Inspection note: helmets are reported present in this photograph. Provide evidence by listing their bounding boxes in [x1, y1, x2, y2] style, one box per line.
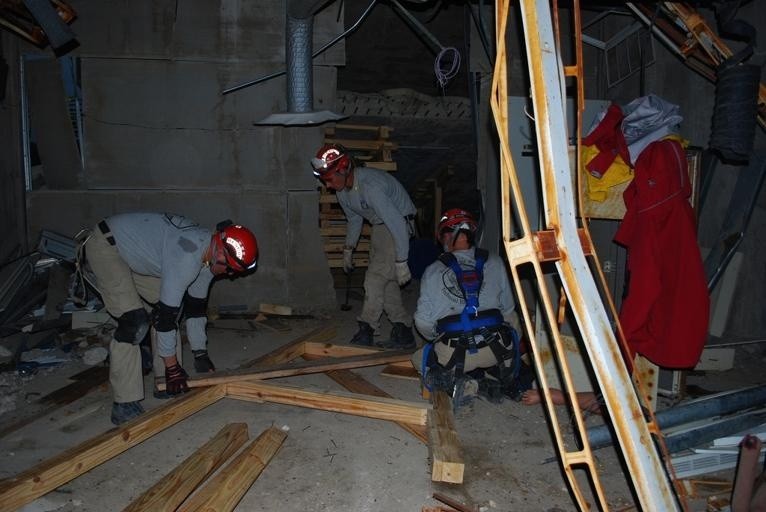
[311, 142, 351, 180]
[215, 224, 259, 276]
[435, 207, 479, 245]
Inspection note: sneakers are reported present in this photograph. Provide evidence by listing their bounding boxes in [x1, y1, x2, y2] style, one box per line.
[111, 401, 146, 425]
[451, 375, 481, 426]
[153, 383, 174, 399]
[477, 379, 505, 404]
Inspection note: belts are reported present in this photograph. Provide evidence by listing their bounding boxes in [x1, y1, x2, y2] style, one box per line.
[405, 213, 415, 222]
[97, 218, 116, 248]
[439, 334, 501, 350]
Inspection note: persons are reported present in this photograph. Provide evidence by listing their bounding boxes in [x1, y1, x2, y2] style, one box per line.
[522, 388, 605, 416]
[411, 207, 540, 406]
[75, 212, 259, 424]
[310, 142, 420, 353]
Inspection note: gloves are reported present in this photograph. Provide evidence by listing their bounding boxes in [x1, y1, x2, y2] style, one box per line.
[164, 362, 191, 396]
[342, 248, 355, 274]
[192, 354, 216, 374]
[394, 261, 412, 286]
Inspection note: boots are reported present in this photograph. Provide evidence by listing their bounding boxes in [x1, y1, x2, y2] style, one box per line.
[376, 321, 417, 351]
[349, 320, 376, 346]
